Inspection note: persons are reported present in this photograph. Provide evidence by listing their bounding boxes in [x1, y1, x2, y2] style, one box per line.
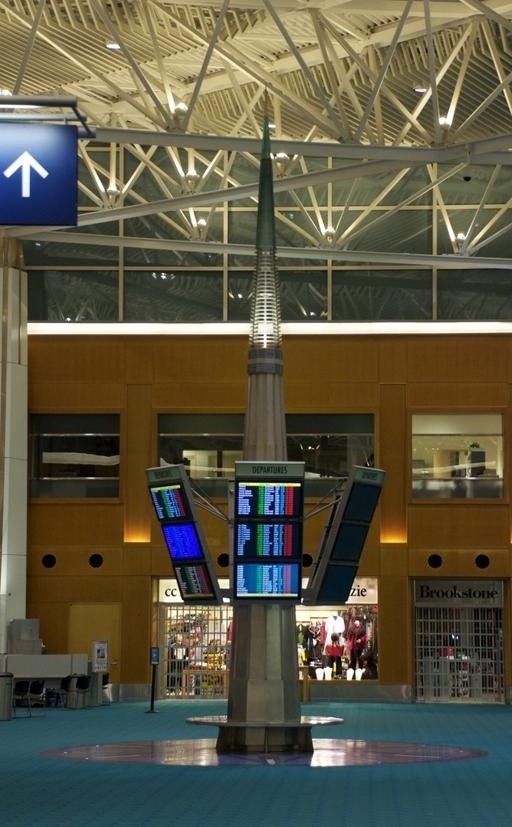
[326, 632, 345, 676]
[322, 613, 346, 668]
[348, 620, 367, 669]
[170, 630, 189, 692]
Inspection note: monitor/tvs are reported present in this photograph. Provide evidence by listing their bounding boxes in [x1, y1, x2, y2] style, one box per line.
[237, 480, 304, 521]
[235, 562, 300, 600]
[345, 483, 382, 523]
[331, 523, 369, 560]
[159, 521, 204, 563]
[169, 559, 215, 599]
[148, 483, 189, 521]
[318, 563, 358, 605]
[235, 523, 300, 559]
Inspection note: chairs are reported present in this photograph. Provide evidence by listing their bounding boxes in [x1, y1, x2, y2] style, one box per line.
[69, 677, 90, 709]
[11, 681, 32, 718]
[49, 677, 71, 708]
[28, 681, 44, 718]
[103, 673, 110, 705]
